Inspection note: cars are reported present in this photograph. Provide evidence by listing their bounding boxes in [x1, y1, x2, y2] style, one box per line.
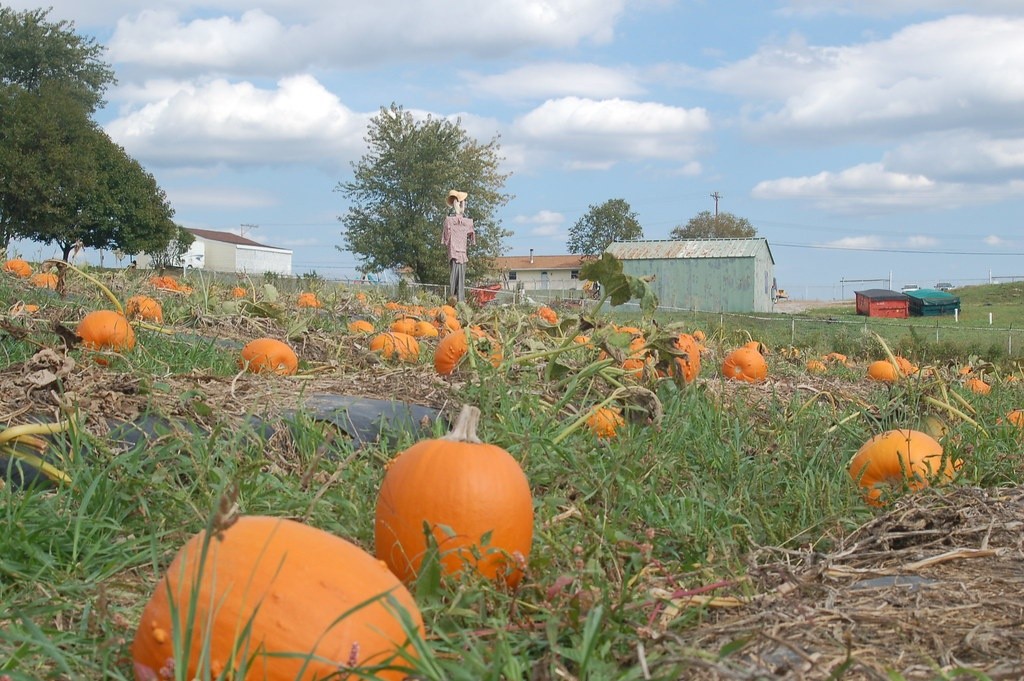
[935, 281, 956, 293]
[901, 284, 921, 295]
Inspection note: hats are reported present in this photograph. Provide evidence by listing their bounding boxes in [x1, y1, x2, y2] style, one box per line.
[445, 189, 468, 207]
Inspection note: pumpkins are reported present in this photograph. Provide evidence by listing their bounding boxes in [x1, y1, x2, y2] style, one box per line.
[132, 515, 424, 681]
[375, 404, 533, 597]
[1, 257, 1024, 513]
